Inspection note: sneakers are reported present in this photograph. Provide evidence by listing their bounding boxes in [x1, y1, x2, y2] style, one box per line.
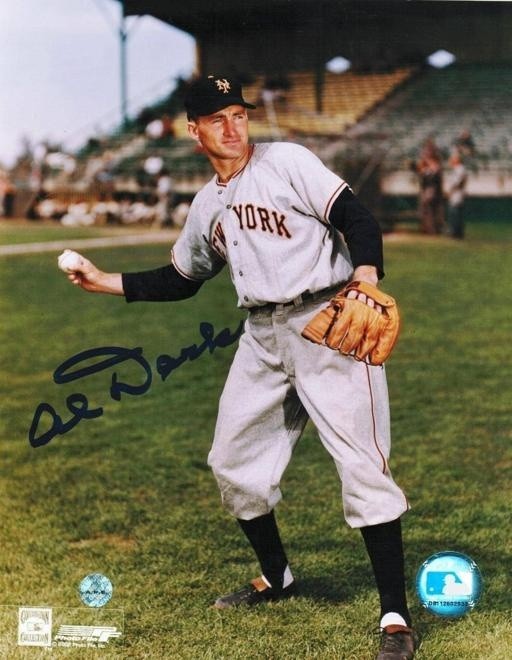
[375, 612, 420, 660]
[216, 575, 298, 612]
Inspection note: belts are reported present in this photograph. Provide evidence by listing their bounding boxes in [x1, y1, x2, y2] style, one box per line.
[247, 283, 348, 313]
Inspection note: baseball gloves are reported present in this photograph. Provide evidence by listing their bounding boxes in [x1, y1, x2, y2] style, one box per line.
[301, 280, 401, 366]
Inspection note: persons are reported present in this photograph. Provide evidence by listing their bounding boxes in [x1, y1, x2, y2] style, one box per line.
[0, 103, 467, 243]
[57, 73, 425, 660]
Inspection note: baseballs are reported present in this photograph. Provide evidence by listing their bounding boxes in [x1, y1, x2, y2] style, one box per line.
[57, 251, 81, 274]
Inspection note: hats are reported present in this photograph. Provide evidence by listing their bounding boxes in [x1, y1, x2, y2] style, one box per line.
[183, 72, 257, 122]
